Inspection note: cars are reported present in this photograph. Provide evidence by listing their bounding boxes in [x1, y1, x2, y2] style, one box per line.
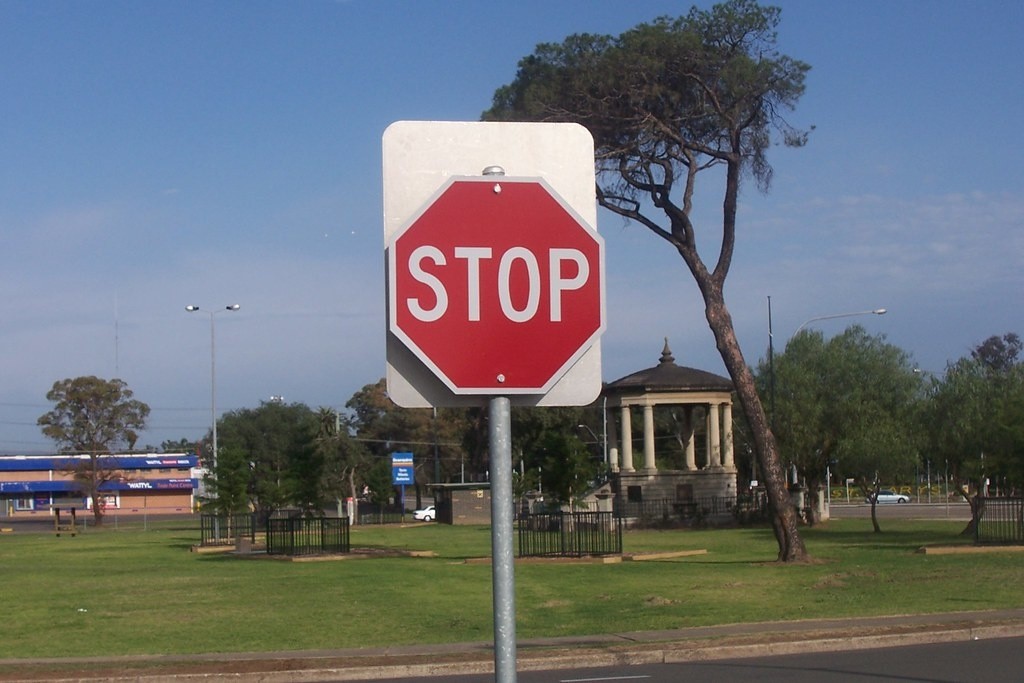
[412, 505, 436, 522]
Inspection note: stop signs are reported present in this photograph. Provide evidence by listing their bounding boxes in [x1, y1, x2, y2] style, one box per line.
[390, 172, 609, 397]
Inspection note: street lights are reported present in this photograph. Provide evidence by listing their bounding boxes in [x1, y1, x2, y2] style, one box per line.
[184, 303, 245, 547]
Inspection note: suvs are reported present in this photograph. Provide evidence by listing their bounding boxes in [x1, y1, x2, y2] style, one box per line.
[865, 490, 911, 503]
